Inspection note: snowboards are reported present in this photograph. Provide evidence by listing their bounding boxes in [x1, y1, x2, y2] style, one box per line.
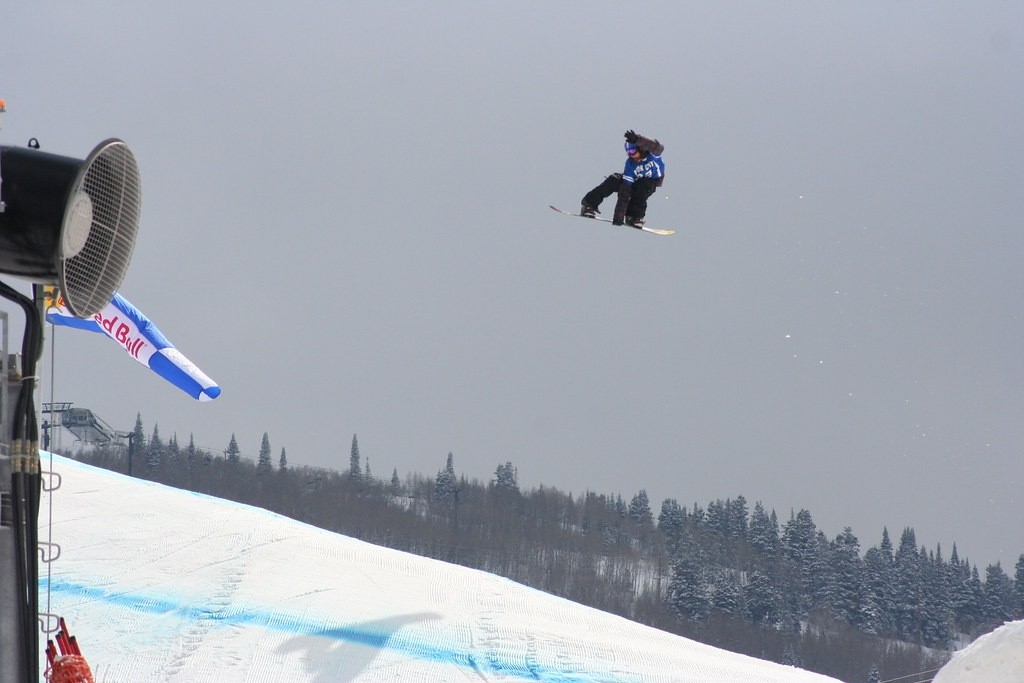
[549, 204, 676, 234]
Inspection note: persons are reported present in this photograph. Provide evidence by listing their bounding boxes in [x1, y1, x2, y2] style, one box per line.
[581, 130, 664, 229]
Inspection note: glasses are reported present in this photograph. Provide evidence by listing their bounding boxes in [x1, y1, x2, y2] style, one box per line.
[626, 149, 637, 155]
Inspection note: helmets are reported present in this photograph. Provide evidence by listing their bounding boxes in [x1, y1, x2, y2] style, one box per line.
[623, 138, 643, 151]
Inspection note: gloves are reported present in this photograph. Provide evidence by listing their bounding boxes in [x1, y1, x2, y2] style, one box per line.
[624, 130, 638, 143]
[613, 214, 624, 225]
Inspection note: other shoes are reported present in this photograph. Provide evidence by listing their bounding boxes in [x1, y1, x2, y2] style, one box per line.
[581, 206, 595, 218]
[626, 216, 644, 225]
